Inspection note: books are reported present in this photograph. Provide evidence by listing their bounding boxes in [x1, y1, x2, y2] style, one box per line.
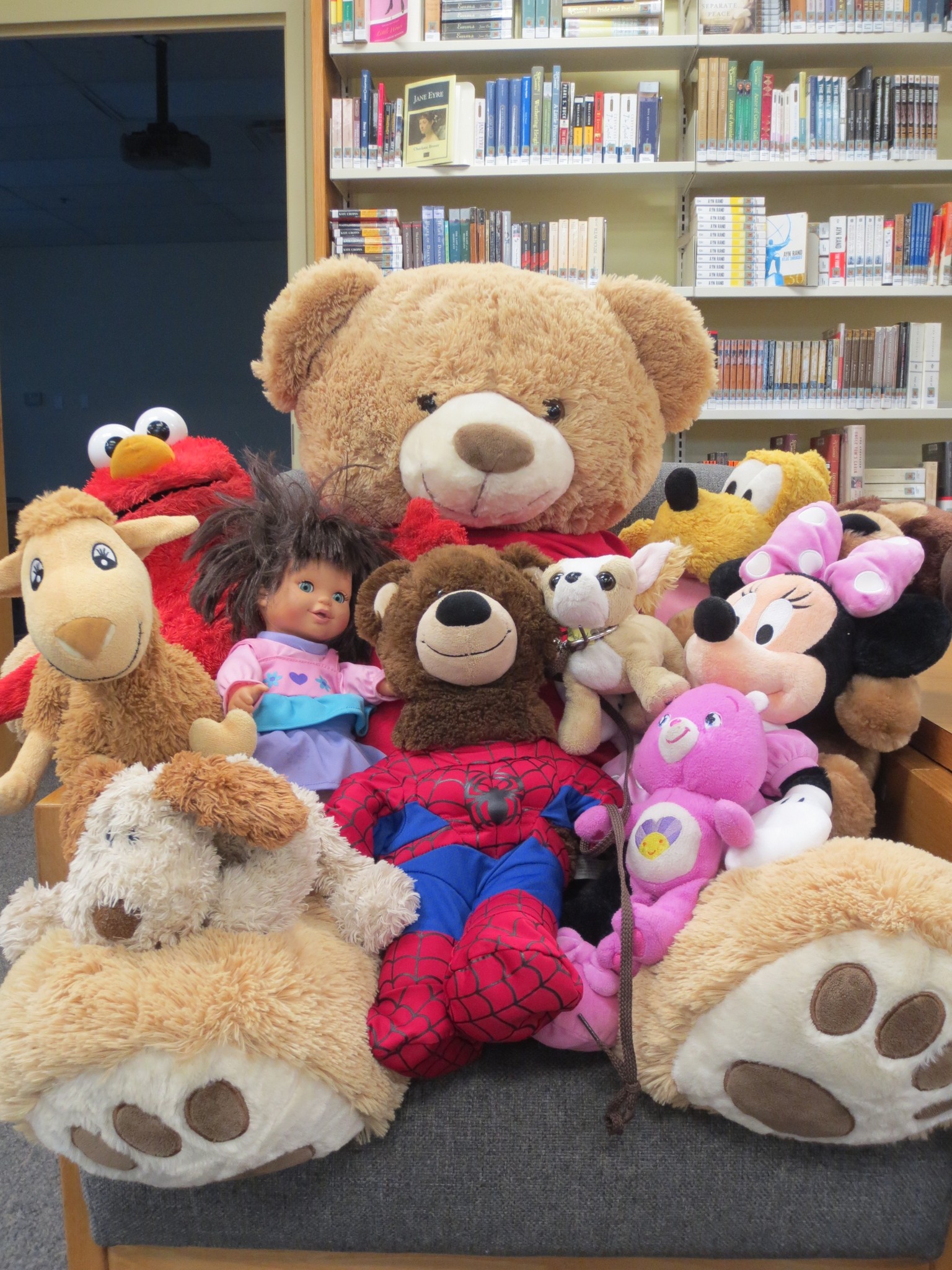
[682, 55, 941, 163]
[328, 0, 952, 43]
[325, 64, 663, 165]
[328, 205, 608, 284]
[687, 196, 952, 290]
[710, 321, 943, 408]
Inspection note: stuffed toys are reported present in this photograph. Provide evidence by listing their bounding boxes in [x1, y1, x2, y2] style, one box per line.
[0, 408, 952, 1192]
[250, 254, 713, 565]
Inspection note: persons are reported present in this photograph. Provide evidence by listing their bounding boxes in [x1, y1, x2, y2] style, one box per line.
[184, 452, 400, 801]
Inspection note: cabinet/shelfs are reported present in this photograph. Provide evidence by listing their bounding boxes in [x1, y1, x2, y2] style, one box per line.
[311, 0, 952, 505]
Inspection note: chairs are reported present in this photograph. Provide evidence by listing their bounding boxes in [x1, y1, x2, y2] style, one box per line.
[31, 723, 952, 1270]
